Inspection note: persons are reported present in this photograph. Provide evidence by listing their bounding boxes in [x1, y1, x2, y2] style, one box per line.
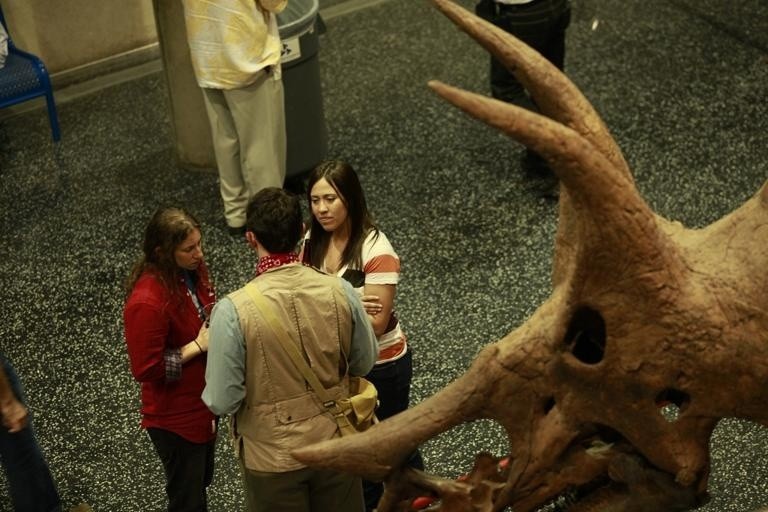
[0, 354, 64, 511]
[181, 0, 289, 237]
[299, 160, 426, 511]
[122, 205, 219, 512]
[202, 187, 380, 511]
[475, 1, 572, 190]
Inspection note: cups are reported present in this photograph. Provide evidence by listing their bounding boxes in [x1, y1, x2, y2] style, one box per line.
[201, 301, 217, 326]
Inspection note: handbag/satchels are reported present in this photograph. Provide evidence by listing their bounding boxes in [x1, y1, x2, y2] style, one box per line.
[330, 375, 381, 437]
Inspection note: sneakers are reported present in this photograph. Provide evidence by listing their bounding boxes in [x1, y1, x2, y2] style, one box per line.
[228, 225, 247, 236]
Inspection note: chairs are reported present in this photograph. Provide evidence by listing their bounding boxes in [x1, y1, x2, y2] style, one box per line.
[0, 2, 60, 143]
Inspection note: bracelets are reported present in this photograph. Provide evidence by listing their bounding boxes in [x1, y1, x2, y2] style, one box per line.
[194, 339, 203, 353]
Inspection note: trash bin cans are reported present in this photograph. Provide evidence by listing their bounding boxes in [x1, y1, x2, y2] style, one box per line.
[275, 0, 327, 178]
